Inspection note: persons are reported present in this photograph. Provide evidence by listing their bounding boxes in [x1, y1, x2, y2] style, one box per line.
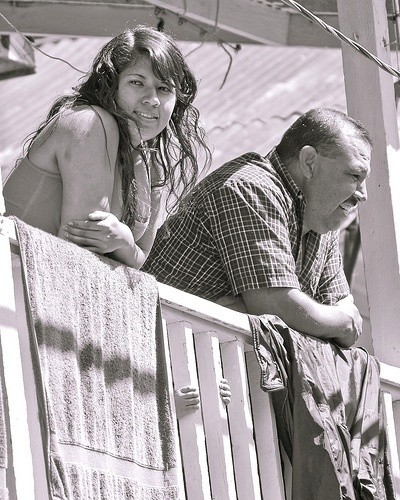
[140, 105, 373, 347]
[2, 28, 212, 270]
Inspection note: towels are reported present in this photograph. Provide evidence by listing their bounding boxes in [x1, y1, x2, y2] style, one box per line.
[8, 215, 181, 500]
